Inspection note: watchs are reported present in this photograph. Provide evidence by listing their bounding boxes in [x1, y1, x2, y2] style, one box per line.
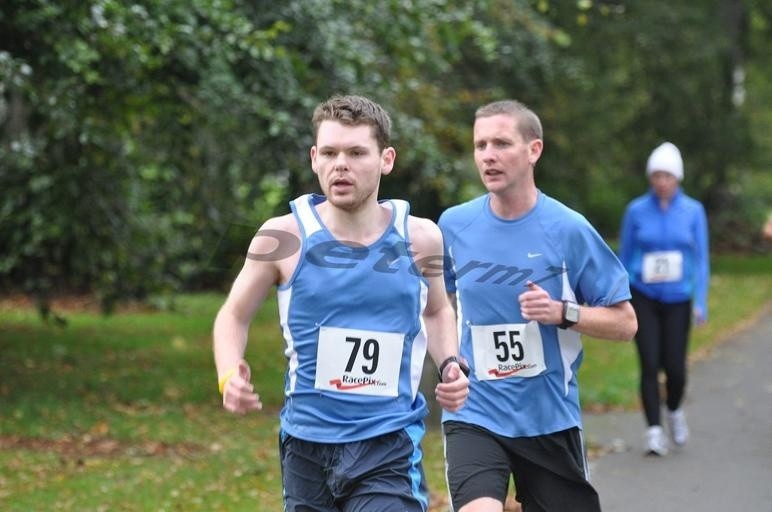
[559, 297, 580, 330]
[437, 357, 471, 383]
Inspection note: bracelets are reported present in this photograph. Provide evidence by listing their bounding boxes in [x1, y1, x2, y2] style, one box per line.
[218, 370, 238, 395]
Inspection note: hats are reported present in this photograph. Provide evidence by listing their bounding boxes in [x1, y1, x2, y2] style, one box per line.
[645, 141, 686, 184]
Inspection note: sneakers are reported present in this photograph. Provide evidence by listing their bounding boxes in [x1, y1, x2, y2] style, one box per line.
[641, 423, 670, 458]
[666, 405, 691, 445]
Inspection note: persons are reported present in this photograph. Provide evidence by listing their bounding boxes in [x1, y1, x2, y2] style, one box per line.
[619, 142, 710, 456]
[212, 94, 471, 512]
[432, 98, 639, 512]
